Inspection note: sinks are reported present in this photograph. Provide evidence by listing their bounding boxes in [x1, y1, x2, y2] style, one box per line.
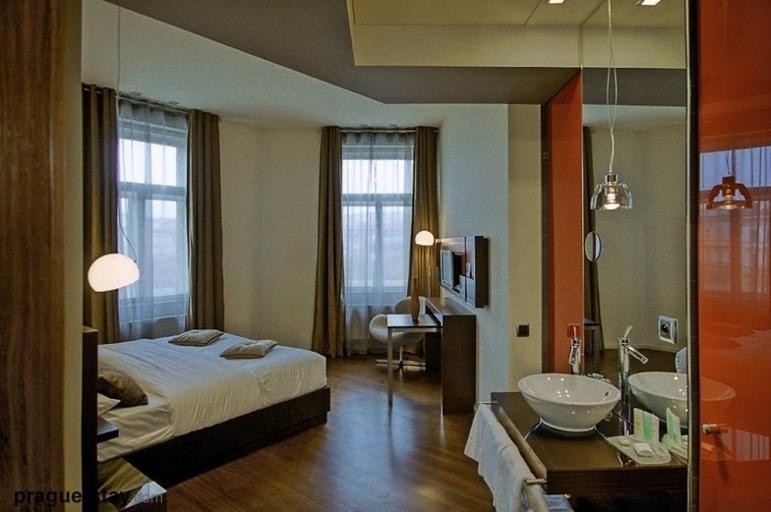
[626, 370, 737, 427]
[516, 372, 622, 431]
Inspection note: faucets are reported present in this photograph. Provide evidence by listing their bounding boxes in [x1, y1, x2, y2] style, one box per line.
[616, 324, 649, 406]
[567, 326, 582, 375]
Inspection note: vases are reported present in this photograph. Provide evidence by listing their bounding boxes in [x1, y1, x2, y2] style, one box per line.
[410, 278, 421, 322]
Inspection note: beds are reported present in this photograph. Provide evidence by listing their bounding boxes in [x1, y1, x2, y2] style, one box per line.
[82, 326, 331, 489]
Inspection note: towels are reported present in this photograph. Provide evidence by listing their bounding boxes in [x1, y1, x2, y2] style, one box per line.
[463, 404, 547, 512]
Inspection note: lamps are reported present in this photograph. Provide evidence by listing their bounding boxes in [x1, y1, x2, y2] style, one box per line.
[87, 7, 140, 293]
[415, 230, 465, 248]
[590, 0, 633, 211]
[705, 0, 754, 211]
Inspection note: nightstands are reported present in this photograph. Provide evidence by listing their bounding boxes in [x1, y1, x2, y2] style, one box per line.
[97, 457, 167, 512]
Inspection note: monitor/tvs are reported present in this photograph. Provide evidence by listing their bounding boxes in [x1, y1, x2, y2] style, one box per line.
[440, 250, 462, 290]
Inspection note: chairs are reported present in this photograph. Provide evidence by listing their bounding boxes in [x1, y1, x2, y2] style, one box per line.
[368, 296, 426, 377]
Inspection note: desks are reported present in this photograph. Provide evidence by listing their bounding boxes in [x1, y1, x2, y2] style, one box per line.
[424, 297, 477, 415]
[386, 314, 440, 407]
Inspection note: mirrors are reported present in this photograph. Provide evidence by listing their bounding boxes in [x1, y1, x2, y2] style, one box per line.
[582, 0, 690, 462]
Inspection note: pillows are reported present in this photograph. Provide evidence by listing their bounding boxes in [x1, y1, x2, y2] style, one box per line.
[219, 339, 280, 358]
[167, 328, 224, 345]
[97, 364, 148, 407]
[97, 392, 121, 417]
